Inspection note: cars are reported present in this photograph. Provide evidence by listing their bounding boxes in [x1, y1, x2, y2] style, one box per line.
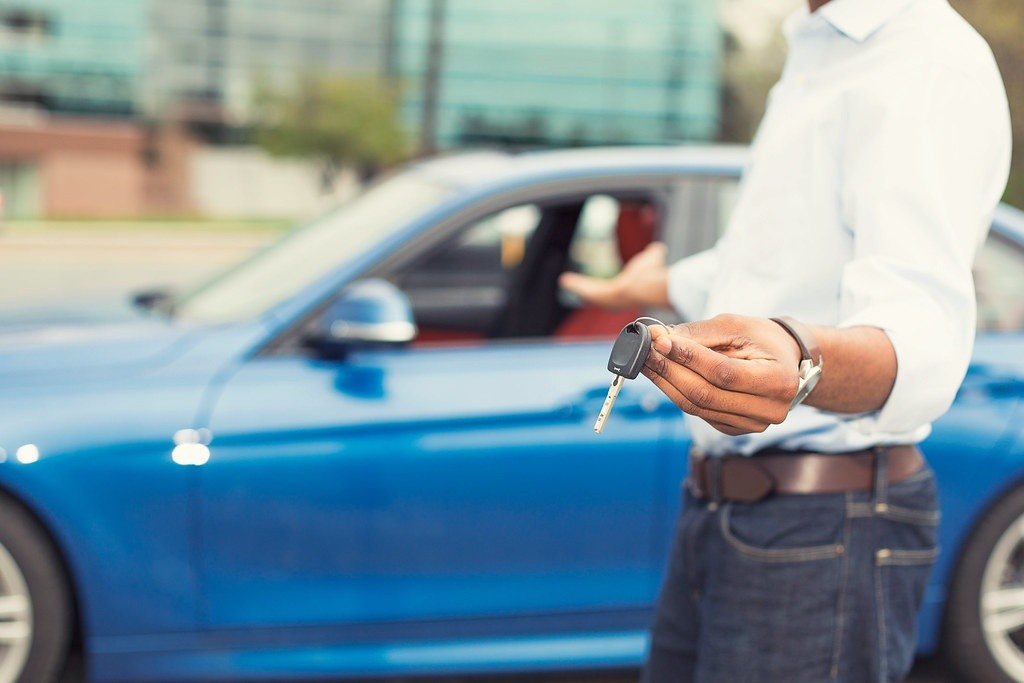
[1, 139, 1024, 683]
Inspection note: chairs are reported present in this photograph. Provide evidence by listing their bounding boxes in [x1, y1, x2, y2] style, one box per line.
[555, 202, 658, 336]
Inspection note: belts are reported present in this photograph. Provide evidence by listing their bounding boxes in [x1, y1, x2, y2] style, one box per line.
[690, 444, 924, 504]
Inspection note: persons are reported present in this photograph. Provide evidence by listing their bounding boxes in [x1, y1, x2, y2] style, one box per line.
[555, 1, 1013, 683]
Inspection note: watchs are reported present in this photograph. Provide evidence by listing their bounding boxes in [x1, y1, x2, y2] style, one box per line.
[766, 316, 825, 412]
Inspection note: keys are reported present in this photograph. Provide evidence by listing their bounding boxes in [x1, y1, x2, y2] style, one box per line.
[595, 320, 653, 434]
